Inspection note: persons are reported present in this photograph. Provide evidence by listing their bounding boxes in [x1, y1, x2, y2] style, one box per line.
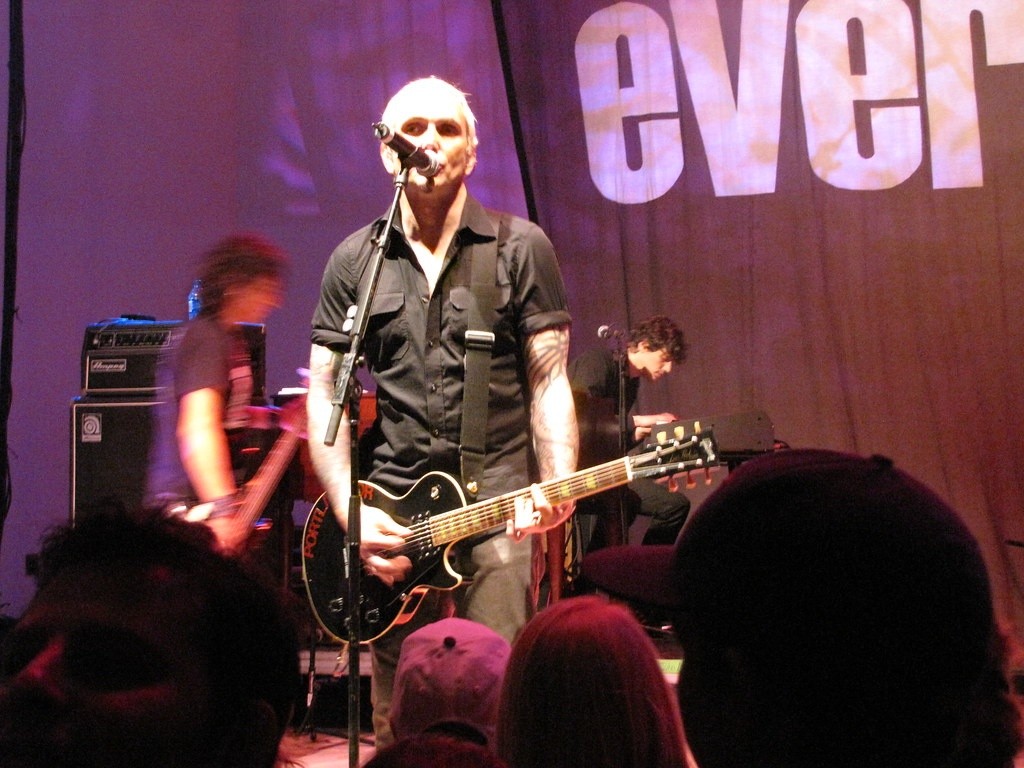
[566, 313, 694, 551]
[361, 595, 691, 767]
[583, 445, 1021, 767]
[0, 506, 301, 768]
[143, 232, 293, 554]
[305, 76, 580, 755]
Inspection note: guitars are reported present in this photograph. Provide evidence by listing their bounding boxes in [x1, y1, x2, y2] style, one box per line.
[218, 400, 306, 553]
[302, 419, 720, 647]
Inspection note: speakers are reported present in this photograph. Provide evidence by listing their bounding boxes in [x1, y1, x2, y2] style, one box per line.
[72, 396, 296, 594]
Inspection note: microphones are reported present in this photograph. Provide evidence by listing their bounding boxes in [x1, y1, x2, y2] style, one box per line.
[371, 122, 440, 177]
[597, 325, 613, 339]
